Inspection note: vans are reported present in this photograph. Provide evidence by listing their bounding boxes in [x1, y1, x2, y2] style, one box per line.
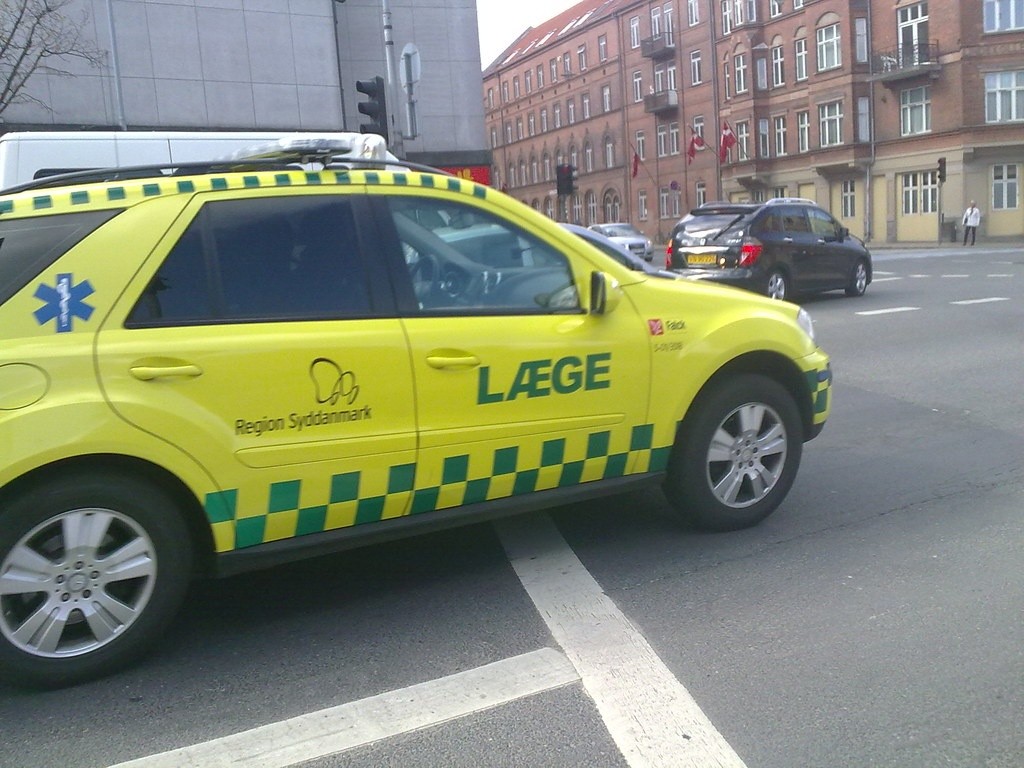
[1, 130, 451, 235]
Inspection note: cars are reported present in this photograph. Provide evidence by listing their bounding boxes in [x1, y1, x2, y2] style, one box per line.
[588, 221, 655, 262]
[414, 222, 678, 304]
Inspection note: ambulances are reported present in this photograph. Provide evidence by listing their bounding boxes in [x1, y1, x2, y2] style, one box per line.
[1, 148, 835, 692]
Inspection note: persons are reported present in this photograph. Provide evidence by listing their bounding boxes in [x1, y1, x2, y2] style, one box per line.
[962, 199, 981, 245]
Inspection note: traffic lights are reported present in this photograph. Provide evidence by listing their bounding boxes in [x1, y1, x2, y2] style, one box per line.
[935, 155, 947, 184]
[355, 73, 390, 146]
[557, 164, 575, 195]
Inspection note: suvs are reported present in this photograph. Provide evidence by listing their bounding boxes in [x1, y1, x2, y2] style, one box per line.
[664, 198, 874, 302]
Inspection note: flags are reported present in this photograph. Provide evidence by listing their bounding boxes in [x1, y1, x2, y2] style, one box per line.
[687, 128, 704, 165]
[633, 152, 640, 178]
[720, 121, 736, 162]
